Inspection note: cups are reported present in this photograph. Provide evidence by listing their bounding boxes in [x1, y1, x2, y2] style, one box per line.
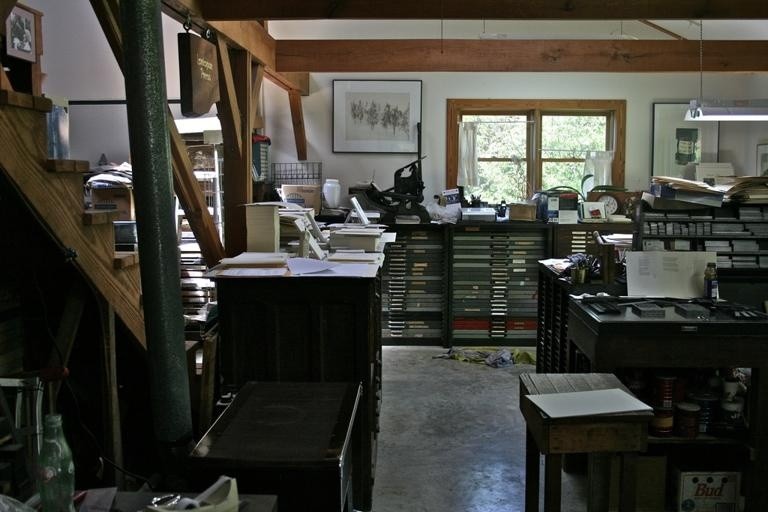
[572, 266, 591, 283]
[722, 378, 738, 400]
[592, 244, 615, 287]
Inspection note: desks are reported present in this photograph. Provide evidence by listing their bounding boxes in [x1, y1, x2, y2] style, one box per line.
[567, 288, 767, 511]
[20, 480, 284, 512]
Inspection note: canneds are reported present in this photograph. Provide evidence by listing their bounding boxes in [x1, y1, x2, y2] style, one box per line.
[626, 373, 718, 439]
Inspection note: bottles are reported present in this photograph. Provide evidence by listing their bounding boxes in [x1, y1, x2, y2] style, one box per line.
[703, 261, 718, 301]
[322, 178, 341, 209]
[36, 413, 77, 512]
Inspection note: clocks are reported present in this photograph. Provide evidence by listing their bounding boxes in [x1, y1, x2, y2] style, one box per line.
[598, 191, 621, 217]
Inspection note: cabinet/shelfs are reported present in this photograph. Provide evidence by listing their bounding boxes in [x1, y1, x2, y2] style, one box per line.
[380, 219, 448, 352]
[534, 250, 628, 379]
[551, 220, 639, 270]
[630, 195, 767, 282]
[448, 215, 550, 352]
[216, 247, 388, 511]
[168, 123, 228, 314]
[182, 375, 372, 509]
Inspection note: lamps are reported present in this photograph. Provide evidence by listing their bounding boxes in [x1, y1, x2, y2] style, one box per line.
[677, 16, 768, 123]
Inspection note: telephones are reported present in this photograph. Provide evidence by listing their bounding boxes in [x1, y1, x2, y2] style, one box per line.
[298, 229, 310, 259]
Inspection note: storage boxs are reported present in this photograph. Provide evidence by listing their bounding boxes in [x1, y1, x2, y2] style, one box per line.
[83, 180, 137, 222]
[507, 201, 540, 224]
[278, 181, 324, 216]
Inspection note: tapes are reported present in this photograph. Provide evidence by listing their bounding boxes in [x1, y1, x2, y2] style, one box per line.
[175, 494, 200, 510]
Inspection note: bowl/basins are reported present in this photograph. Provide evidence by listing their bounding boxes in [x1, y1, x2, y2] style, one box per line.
[509, 203, 538, 221]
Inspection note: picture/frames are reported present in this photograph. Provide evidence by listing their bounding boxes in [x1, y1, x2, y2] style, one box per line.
[648, 99, 722, 189]
[330, 76, 425, 156]
[756, 141, 767, 181]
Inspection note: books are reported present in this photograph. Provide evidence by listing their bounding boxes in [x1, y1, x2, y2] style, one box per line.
[523, 388, 653, 419]
[602, 175, 768, 269]
[177, 209, 383, 340]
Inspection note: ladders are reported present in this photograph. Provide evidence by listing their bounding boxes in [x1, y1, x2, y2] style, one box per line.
[173, 148, 225, 274]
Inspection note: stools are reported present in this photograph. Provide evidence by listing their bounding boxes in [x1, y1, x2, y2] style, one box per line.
[513, 373, 662, 510]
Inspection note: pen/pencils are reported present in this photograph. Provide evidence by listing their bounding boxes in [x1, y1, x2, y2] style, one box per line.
[574, 255, 599, 268]
[471, 194, 481, 201]
